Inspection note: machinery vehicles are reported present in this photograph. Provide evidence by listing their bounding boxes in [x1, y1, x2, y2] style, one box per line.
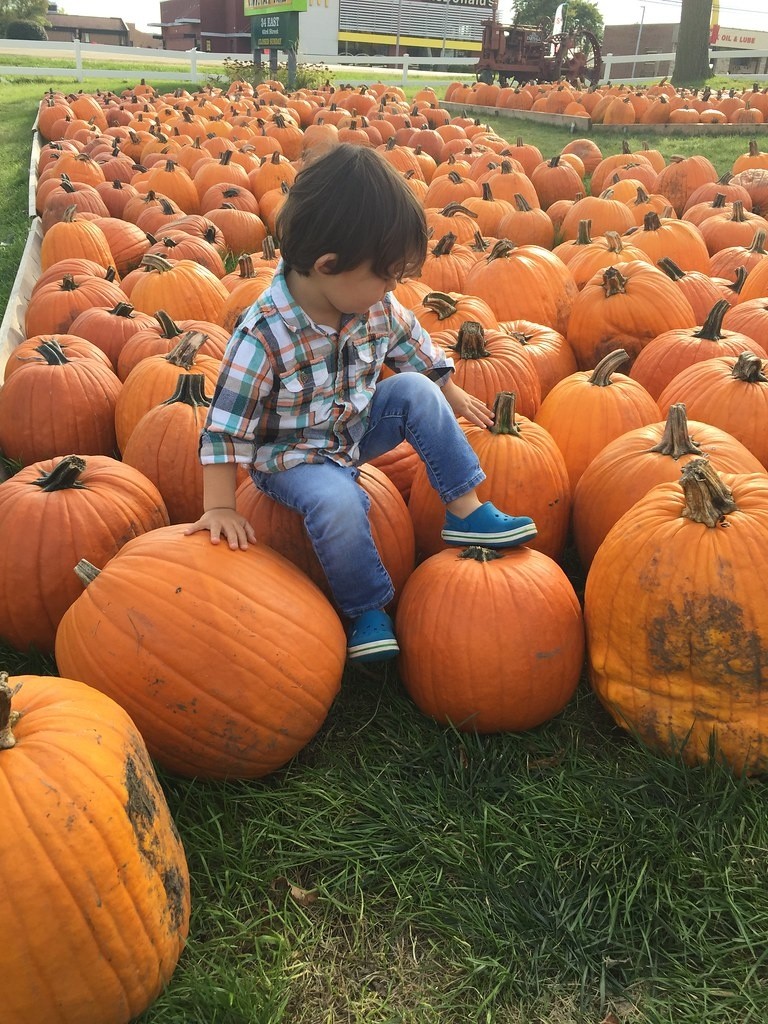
[474, 2, 603, 93]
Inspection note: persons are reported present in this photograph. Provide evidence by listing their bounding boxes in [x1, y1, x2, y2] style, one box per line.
[183, 140, 538, 664]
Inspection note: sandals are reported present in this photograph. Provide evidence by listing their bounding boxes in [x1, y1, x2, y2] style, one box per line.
[346, 609, 402, 664]
[441, 501, 541, 551]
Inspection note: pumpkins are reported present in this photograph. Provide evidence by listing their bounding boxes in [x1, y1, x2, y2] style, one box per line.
[0, 674, 192, 1024]
[444, 76, 768, 124]
[0, 139, 768, 781]
[26, 80, 544, 213]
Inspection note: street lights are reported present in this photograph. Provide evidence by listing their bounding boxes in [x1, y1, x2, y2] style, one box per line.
[628, 5, 647, 78]
